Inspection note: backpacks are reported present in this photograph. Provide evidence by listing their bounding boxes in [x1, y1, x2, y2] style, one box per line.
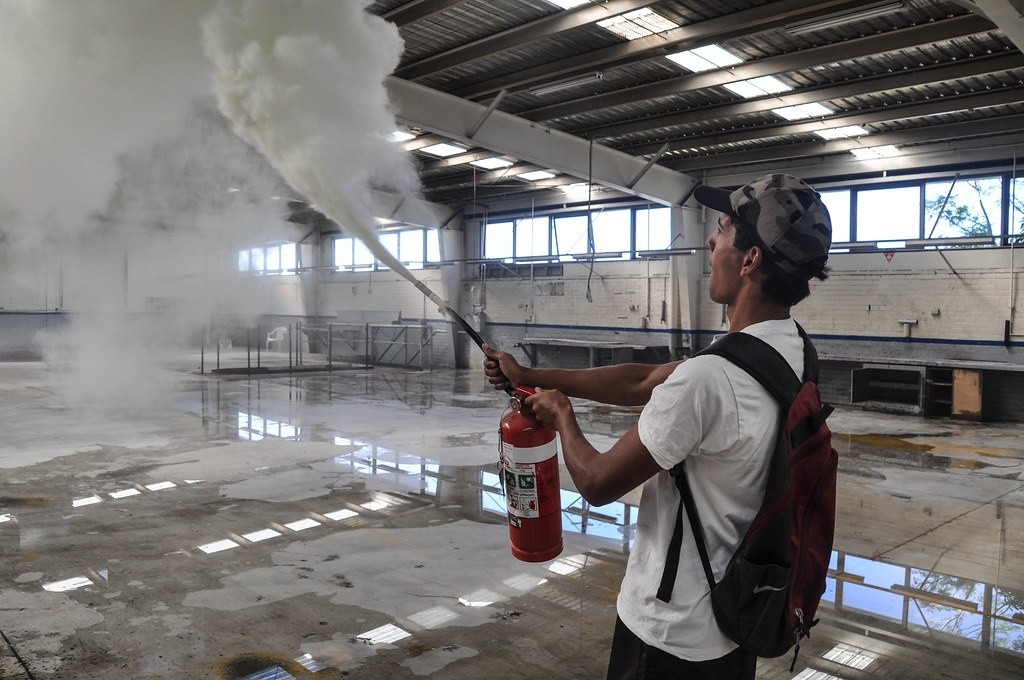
[655, 319, 839, 671]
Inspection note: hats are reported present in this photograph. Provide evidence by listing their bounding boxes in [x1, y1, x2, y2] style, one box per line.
[694, 173, 832, 280]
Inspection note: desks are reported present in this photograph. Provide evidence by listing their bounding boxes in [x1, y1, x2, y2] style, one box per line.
[818, 354, 1024, 423]
[501, 338, 646, 369]
[328, 323, 433, 371]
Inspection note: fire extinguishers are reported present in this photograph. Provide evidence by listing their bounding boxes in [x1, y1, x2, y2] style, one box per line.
[413, 279, 563, 562]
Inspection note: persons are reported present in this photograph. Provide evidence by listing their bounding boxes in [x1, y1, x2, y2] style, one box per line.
[481, 173, 833, 680]
